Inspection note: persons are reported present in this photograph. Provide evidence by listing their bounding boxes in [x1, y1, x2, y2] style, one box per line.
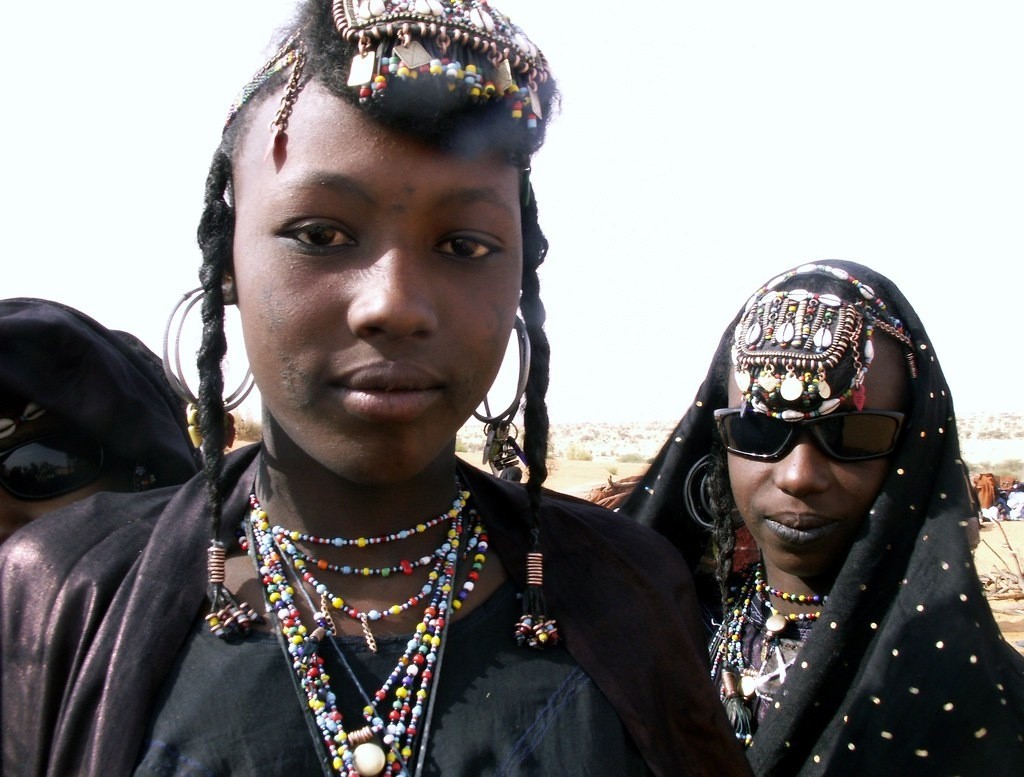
[619, 259, 1024, 776]
[0, 0, 750, 777]
[0, 301, 202, 543]
[980, 483, 1024, 522]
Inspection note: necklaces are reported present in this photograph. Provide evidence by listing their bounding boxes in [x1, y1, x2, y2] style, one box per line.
[240, 481, 488, 776]
[709, 563, 828, 743]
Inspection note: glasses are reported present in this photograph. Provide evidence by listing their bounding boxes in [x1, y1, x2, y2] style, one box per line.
[714, 408, 908, 464]
